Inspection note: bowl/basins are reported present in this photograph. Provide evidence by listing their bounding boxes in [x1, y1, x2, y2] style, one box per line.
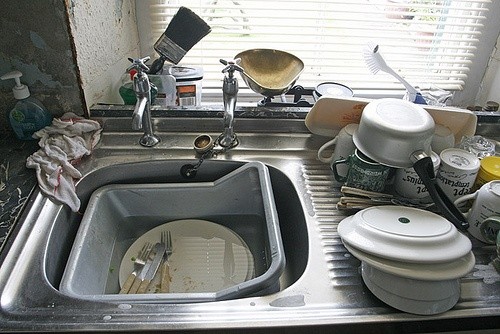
[361, 260, 460, 301]
[234, 49, 305, 96]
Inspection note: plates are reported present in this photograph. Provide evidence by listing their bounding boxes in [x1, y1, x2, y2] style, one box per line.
[337, 206, 473, 263]
[220, 225, 254, 288]
[359, 270, 460, 316]
[119, 219, 248, 294]
[340, 239, 475, 281]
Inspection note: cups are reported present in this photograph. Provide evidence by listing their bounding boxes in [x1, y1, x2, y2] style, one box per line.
[453, 179, 500, 244]
[480, 215, 500, 272]
[332, 147, 388, 197]
[317, 124, 358, 176]
[438, 146, 480, 209]
[431, 123, 456, 153]
[387, 150, 441, 199]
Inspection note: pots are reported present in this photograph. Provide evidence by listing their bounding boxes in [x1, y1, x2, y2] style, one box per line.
[352, 98, 469, 229]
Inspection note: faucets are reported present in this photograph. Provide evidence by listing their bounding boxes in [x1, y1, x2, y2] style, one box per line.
[125, 55, 160, 147]
[216, 56, 245, 149]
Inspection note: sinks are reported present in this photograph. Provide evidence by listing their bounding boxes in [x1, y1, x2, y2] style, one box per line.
[40, 157, 312, 313]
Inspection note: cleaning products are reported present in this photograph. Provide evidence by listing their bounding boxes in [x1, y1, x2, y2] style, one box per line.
[1, 70, 47, 139]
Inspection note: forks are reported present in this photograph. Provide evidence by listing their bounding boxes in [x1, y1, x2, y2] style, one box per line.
[160, 231, 172, 293]
[119, 242, 155, 294]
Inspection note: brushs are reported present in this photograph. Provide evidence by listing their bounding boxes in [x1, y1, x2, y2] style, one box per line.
[147, 5, 211, 74]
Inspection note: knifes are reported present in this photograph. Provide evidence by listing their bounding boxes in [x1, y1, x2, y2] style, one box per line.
[127, 243, 161, 294]
[137, 244, 165, 293]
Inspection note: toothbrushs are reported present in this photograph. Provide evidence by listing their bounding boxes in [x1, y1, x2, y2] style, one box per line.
[363, 42, 433, 104]
